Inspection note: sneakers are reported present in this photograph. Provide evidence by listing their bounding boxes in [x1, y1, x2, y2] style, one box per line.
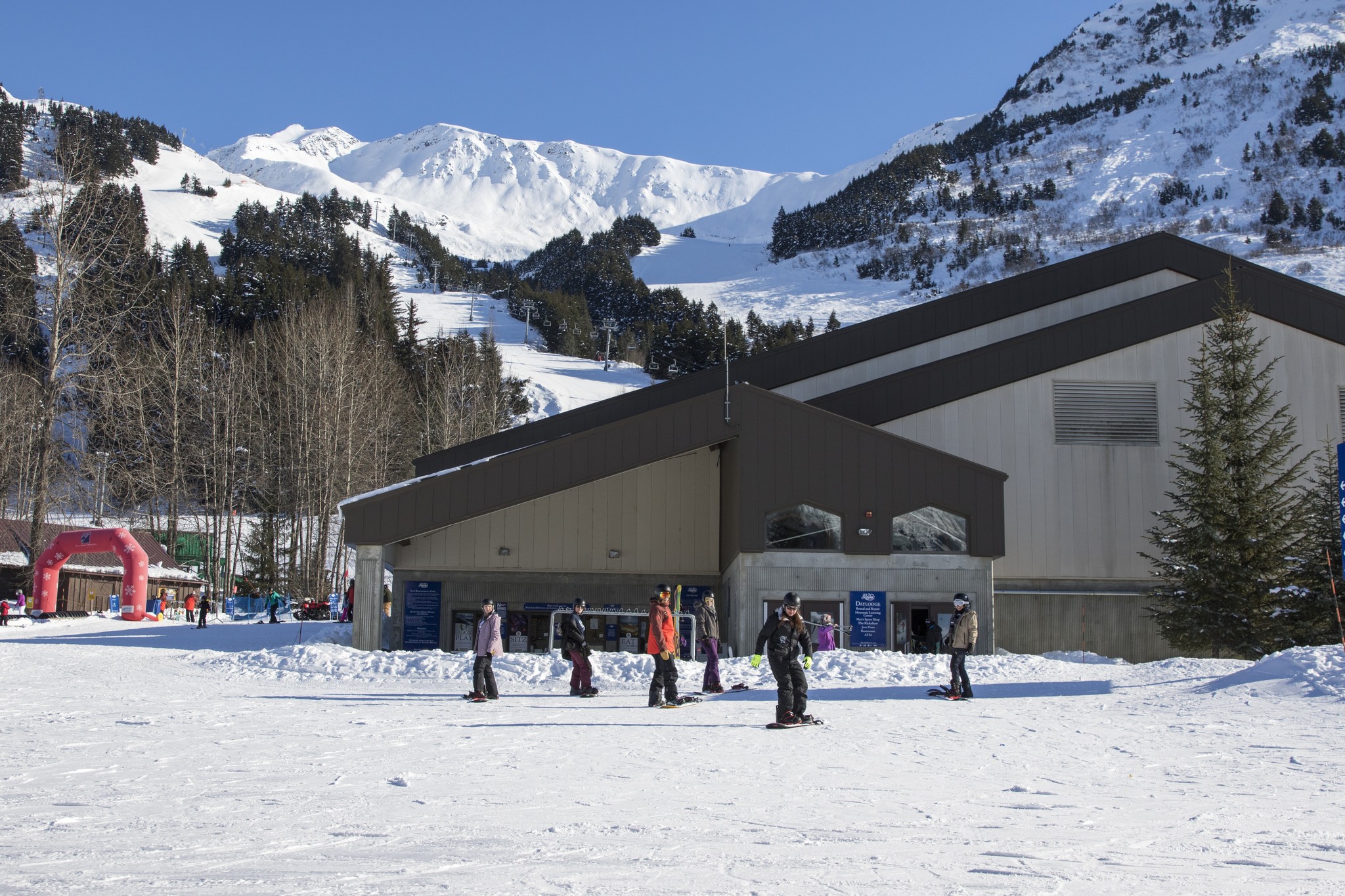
[570, 688, 581, 695]
[712, 682, 723, 692]
[779, 711, 802, 724]
[197, 625, 202, 629]
[666, 697, 685, 706]
[800, 714, 814, 723]
[202, 625, 206, 628]
[702, 686, 712, 692]
[649, 701, 666, 707]
[469, 691, 499, 699]
[581, 686, 599, 695]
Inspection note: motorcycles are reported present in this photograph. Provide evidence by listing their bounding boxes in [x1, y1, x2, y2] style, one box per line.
[293, 598, 332, 621]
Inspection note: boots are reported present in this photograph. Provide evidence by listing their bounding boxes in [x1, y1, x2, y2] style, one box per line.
[961, 680, 973, 698]
[949, 680, 961, 696]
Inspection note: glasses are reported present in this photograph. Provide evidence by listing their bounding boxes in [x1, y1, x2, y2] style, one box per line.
[704, 599, 713, 602]
[786, 605, 798, 610]
[953, 601, 969, 606]
[576, 605, 584, 609]
[659, 592, 670, 598]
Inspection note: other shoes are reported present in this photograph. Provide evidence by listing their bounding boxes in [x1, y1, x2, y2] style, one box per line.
[339, 620, 344, 622]
[269, 620, 277, 623]
[348, 621, 353, 622]
[0, 624, 4, 626]
[4, 624, 8, 626]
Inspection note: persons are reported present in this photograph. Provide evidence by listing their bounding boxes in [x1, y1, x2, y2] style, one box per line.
[197, 596, 211, 629]
[14, 589, 27, 615]
[472, 598, 503, 699]
[383, 584, 392, 617]
[157, 588, 168, 619]
[184, 590, 197, 623]
[817, 614, 836, 651]
[695, 591, 723, 693]
[923, 619, 944, 654]
[557, 597, 598, 696]
[345, 579, 355, 622]
[647, 584, 685, 706]
[945, 593, 978, 698]
[750, 592, 814, 725]
[340, 592, 349, 622]
[265, 587, 287, 623]
[0, 598, 10, 626]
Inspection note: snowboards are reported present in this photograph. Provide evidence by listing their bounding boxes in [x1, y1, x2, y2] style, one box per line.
[926, 689, 968, 701]
[765, 722, 815, 728]
[802, 619, 852, 637]
[463, 694, 487, 702]
[693, 688, 757, 695]
[660, 697, 704, 708]
[579, 689, 602, 698]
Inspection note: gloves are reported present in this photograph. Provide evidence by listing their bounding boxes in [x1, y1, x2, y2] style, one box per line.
[966, 642, 974, 656]
[802, 656, 812, 670]
[582, 641, 586, 648]
[265, 604, 267, 609]
[13, 605, 16, 609]
[750, 654, 761, 668]
[660, 650, 671, 660]
[944, 636, 951, 648]
[717, 640, 721, 650]
[282, 600, 287, 606]
[702, 635, 710, 648]
[485, 652, 492, 659]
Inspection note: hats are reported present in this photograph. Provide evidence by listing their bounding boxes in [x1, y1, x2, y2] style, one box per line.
[821, 613, 831, 623]
[161, 589, 166, 592]
[921, 616, 936, 627]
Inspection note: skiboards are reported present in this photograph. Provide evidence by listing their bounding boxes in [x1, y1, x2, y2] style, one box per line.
[191, 624, 209, 629]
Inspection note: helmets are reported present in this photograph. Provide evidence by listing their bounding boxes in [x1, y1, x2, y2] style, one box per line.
[573, 597, 586, 610]
[384, 584, 389, 588]
[702, 590, 715, 603]
[481, 598, 494, 610]
[783, 592, 801, 610]
[268, 587, 273, 592]
[350, 580, 354, 584]
[953, 593, 968, 605]
[16, 589, 22, 594]
[2, 598, 8, 603]
[654, 584, 671, 600]
